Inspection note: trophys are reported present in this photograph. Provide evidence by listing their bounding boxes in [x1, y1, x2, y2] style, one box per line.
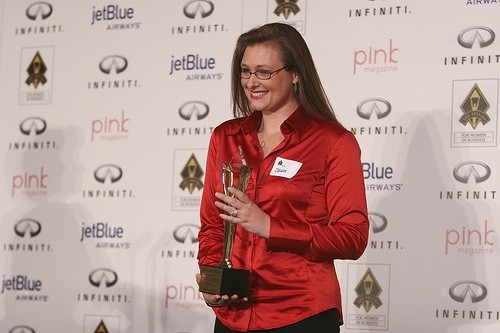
[198, 144, 251, 298]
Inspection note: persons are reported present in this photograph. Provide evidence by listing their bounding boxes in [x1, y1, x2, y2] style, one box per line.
[195, 23, 369, 333]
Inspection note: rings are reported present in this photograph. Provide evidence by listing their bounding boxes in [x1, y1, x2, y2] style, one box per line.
[233, 208, 238, 217]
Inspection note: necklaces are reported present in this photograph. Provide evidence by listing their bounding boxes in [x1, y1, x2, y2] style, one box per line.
[260, 117, 280, 148]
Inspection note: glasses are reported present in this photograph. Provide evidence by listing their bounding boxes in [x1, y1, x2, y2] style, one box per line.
[237, 66, 288, 80]
